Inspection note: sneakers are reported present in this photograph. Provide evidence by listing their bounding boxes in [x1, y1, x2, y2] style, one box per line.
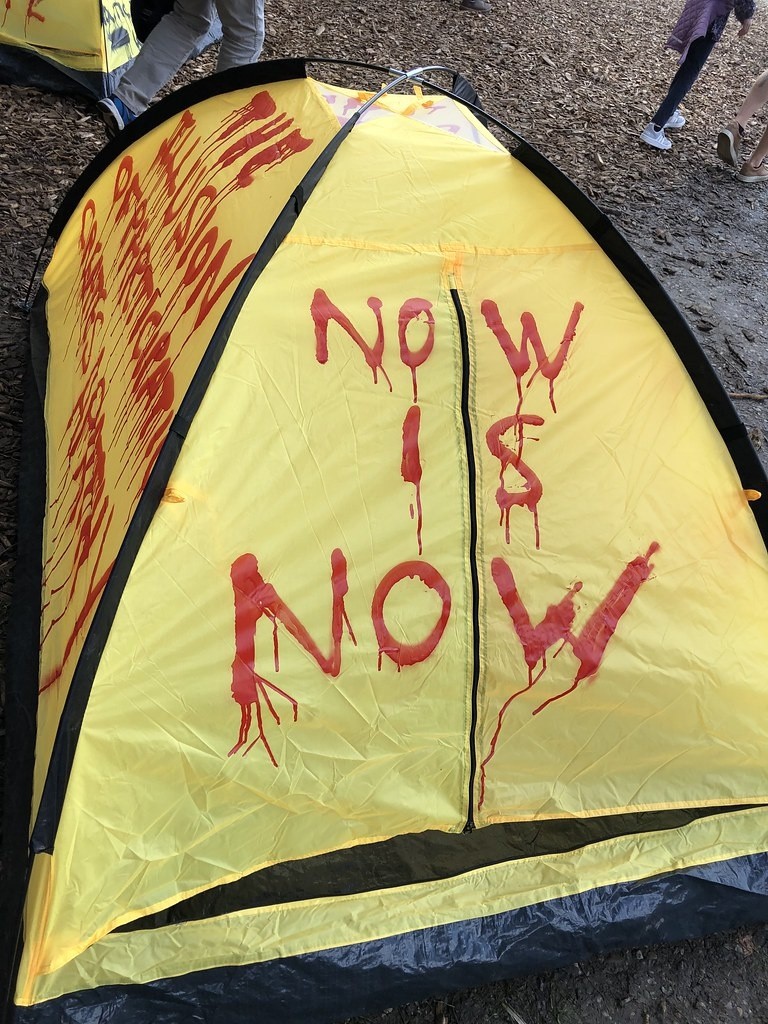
[661, 112, 687, 129]
[96, 95, 137, 141]
[639, 122, 674, 149]
[717, 120, 740, 167]
[736, 163, 768, 182]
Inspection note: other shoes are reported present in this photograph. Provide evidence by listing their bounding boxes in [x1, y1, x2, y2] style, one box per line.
[459, 0, 493, 12]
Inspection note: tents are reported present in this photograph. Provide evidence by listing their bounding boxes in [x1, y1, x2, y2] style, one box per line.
[0, 0, 223, 102]
[1, 57, 767, 1024]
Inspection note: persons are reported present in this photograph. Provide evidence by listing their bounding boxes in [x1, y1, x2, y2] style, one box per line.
[639, 0, 755, 150]
[95, 0, 267, 138]
[716, 68, 768, 183]
